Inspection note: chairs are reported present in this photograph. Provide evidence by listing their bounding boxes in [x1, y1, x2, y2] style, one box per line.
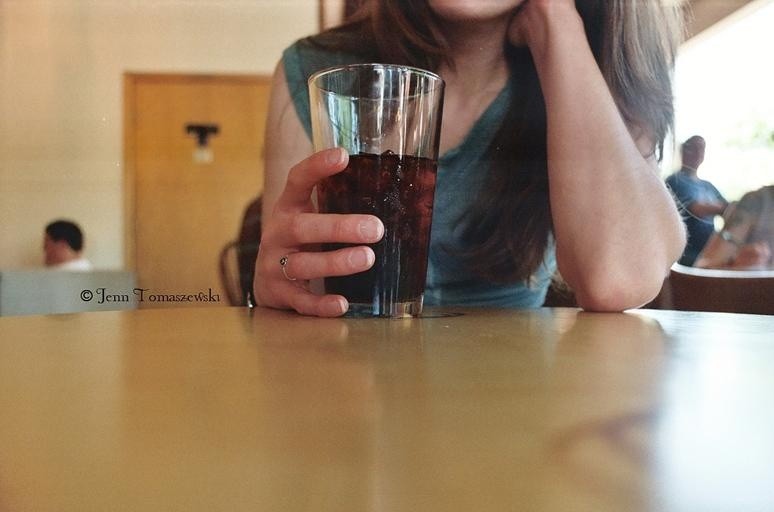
[669, 258, 773, 317]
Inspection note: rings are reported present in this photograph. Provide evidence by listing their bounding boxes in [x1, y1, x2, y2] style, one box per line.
[280, 251, 297, 282]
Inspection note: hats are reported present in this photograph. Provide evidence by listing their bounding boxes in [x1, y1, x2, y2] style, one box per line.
[682, 136, 705, 148]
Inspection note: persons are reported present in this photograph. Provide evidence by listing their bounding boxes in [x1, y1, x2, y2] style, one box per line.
[664, 136, 729, 266]
[42, 217, 92, 273]
[252, 0, 688, 317]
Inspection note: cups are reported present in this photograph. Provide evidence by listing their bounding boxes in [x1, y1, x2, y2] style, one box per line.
[304, 64, 445, 320]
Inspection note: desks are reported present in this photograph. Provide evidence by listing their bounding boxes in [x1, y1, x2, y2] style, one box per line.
[0, 307, 773, 512]
